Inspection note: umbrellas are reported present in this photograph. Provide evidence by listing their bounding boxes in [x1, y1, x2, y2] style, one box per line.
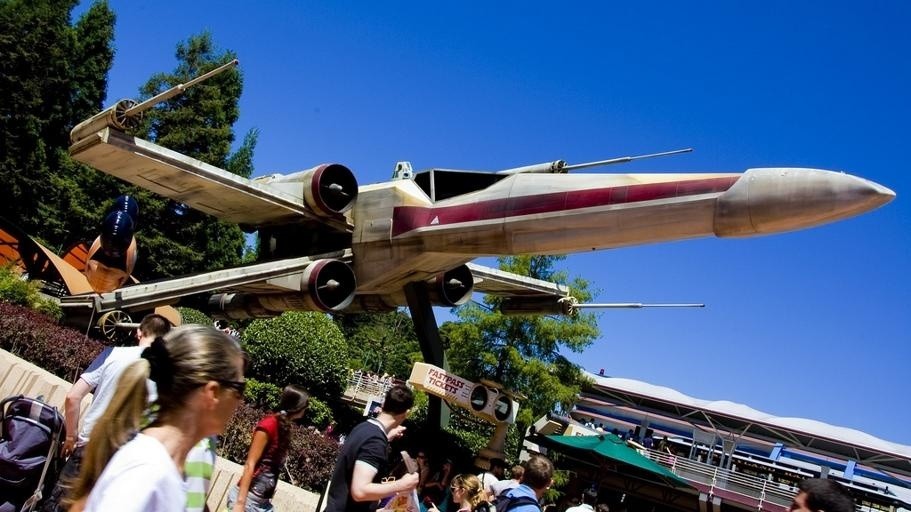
[542, 432, 700, 492]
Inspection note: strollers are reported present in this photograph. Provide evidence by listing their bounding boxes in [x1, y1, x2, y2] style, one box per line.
[0, 394, 69, 512]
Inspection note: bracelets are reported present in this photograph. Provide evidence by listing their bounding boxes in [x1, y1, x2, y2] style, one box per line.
[65, 434, 77, 441]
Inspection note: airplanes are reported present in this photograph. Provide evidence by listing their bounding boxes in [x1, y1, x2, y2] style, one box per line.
[26, 57, 899, 349]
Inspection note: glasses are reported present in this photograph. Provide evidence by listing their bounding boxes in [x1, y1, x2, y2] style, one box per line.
[219, 379, 247, 396]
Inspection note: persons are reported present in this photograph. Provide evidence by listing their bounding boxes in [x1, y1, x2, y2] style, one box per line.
[785, 477, 856, 512]
[346, 368, 397, 398]
[226, 384, 310, 512]
[322, 384, 420, 512]
[59, 322, 248, 512]
[697, 442, 807, 487]
[134, 404, 219, 512]
[577, 416, 672, 456]
[318, 448, 614, 512]
[44, 313, 172, 512]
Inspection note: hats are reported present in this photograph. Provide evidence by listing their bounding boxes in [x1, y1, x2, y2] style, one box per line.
[489, 457, 509, 469]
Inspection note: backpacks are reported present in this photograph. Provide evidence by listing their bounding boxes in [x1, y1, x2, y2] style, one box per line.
[471, 488, 539, 512]
